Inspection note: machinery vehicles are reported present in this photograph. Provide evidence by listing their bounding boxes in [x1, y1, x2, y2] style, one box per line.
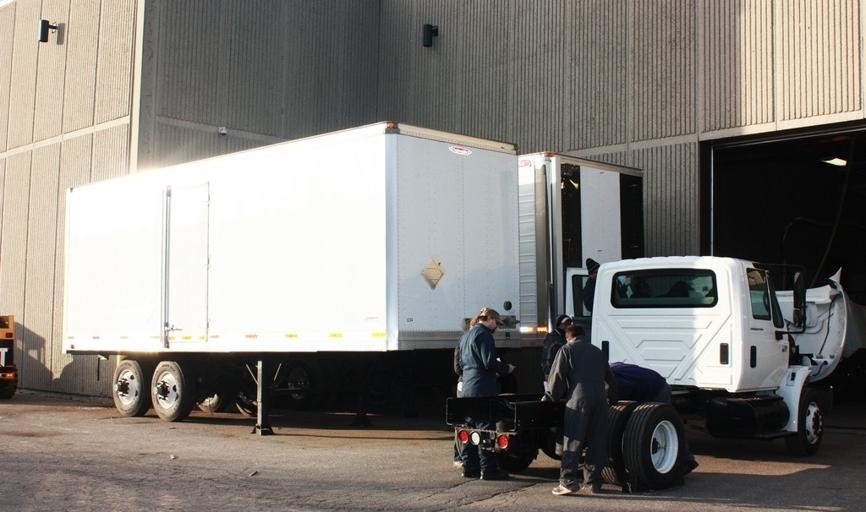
[0, 315, 19, 401]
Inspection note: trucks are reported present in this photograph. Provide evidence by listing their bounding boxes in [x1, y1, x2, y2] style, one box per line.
[443, 254, 830, 489]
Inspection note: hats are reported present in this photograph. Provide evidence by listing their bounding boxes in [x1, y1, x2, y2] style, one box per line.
[554, 313, 571, 329]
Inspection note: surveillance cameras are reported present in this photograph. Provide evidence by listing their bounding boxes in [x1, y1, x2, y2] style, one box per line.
[220, 127, 227, 136]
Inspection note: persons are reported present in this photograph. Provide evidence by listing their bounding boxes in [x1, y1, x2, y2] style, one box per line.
[541, 314, 573, 381]
[459, 308, 519, 481]
[451, 316, 472, 462]
[583, 257, 620, 315]
[541, 324, 619, 497]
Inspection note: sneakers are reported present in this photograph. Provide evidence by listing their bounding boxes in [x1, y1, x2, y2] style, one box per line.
[581, 483, 601, 494]
[552, 484, 571, 495]
[461, 470, 508, 480]
